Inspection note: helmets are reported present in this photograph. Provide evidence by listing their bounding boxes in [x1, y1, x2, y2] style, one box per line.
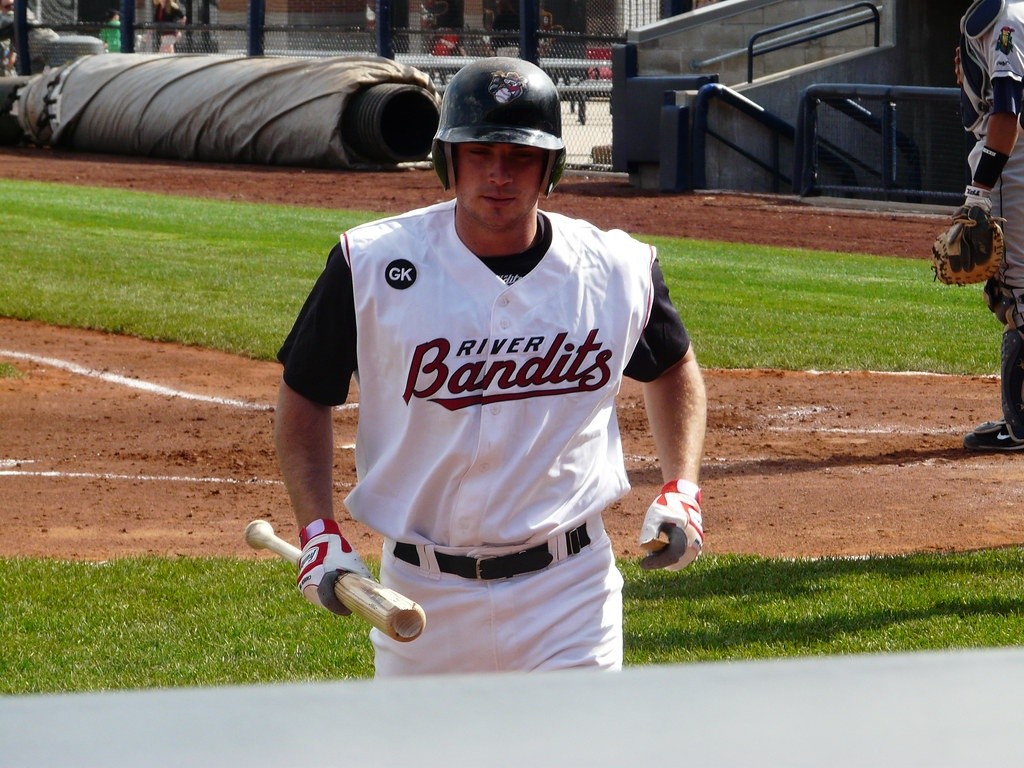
[433, 56, 566, 199]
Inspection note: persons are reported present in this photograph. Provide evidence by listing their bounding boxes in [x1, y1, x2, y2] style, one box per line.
[273, 58, 706, 674]
[931, 0, 1024, 456]
[428, 25, 466, 84]
[548, 25, 570, 86]
[100, 10, 121, 53]
[141, 0, 187, 53]
[0, 0, 17, 76]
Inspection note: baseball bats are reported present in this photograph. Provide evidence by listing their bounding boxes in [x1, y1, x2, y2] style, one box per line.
[243, 518, 428, 644]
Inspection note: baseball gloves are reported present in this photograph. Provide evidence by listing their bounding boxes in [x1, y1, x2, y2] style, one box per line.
[928, 202, 1009, 287]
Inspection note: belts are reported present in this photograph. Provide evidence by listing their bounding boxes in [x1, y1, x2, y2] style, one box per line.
[395, 522, 592, 579]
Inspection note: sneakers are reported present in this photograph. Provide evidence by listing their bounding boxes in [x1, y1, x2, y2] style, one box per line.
[964, 422, 1024, 451]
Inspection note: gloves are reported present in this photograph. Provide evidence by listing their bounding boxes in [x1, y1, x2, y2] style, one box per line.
[295, 518, 372, 614]
[641, 479, 704, 573]
